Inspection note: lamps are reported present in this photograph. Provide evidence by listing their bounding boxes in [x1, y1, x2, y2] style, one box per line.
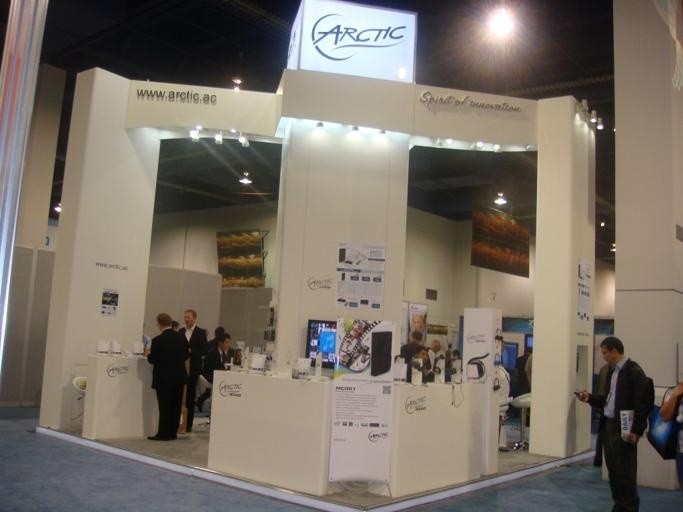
[596, 117, 604, 130]
[214, 133, 224, 146]
[190, 130, 200, 144]
[494, 193, 508, 205]
[240, 137, 250, 147]
[239, 172, 253, 184]
[589, 110, 597, 124]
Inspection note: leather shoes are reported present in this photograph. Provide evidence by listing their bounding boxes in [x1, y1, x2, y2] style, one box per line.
[168, 434, 176, 441]
[146, 434, 171, 441]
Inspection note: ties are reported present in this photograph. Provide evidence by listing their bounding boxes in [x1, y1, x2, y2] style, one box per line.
[222, 350, 225, 369]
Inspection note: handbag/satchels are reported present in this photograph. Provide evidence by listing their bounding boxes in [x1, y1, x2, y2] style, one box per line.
[645, 405, 680, 460]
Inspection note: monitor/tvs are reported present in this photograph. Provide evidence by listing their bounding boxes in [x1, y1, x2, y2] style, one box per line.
[501, 342, 518, 371]
[306, 320, 337, 369]
[524, 334, 533, 355]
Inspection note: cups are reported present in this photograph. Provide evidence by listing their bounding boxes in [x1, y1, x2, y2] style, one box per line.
[292, 368, 299, 378]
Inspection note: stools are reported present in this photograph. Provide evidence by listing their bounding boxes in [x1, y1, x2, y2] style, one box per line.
[69, 375, 88, 422]
[199, 375, 213, 428]
[498, 396, 513, 450]
[512, 393, 532, 450]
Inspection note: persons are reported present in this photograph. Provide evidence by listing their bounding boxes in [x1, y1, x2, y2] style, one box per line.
[193, 332, 232, 412]
[399, 331, 462, 384]
[411, 314, 426, 331]
[515, 346, 532, 426]
[207, 326, 225, 348]
[178, 310, 208, 433]
[574, 337, 651, 512]
[353, 323, 364, 336]
[594, 363, 610, 466]
[172, 320, 180, 330]
[660, 381, 683, 495]
[524, 354, 532, 387]
[143, 313, 190, 441]
